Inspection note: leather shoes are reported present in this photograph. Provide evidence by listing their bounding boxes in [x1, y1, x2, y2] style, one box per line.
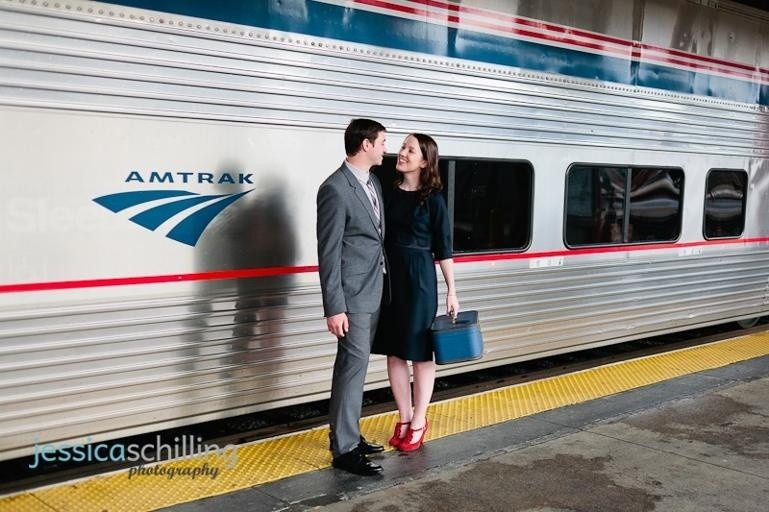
[329, 431, 385, 476]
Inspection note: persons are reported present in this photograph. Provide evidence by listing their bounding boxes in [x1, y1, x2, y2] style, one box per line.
[370, 133, 459, 451]
[316, 119, 391, 476]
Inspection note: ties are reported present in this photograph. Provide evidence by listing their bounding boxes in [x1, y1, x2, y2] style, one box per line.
[366, 180, 382, 232]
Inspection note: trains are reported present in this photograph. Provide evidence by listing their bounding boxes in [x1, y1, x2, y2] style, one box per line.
[0, 1, 768, 462]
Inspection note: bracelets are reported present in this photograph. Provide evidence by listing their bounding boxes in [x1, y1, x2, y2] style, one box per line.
[446, 294, 456, 297]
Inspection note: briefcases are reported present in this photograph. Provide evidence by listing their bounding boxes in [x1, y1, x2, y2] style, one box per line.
[430, 310, 483, 365]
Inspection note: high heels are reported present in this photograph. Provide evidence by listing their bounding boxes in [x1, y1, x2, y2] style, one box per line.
[389, 416, 429, 452]
